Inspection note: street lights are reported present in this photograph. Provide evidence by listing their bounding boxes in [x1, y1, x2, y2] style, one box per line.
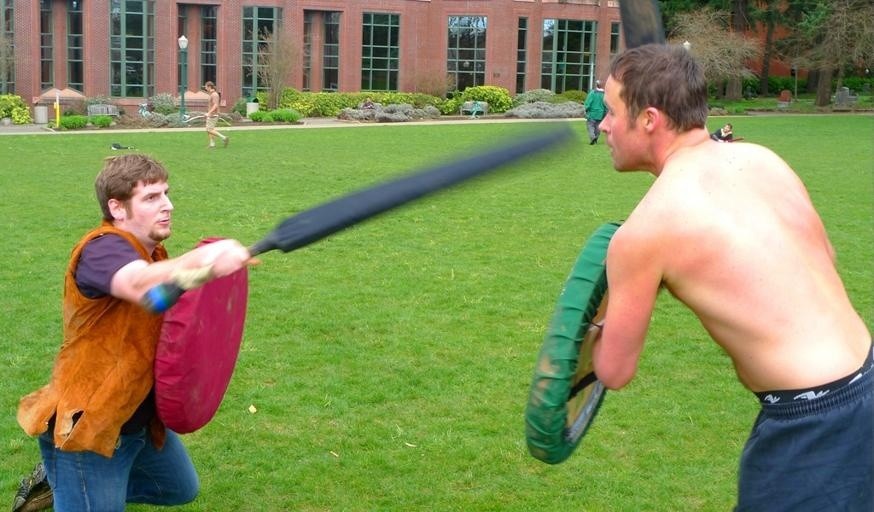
[176, 35, 188, 124]
[681, 41, 689, 54]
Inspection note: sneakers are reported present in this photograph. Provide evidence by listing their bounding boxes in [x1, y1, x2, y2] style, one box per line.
[207, 135, 230, 149]
[12, 461, 53, 511]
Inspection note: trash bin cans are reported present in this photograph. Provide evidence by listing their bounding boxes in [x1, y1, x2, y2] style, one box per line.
[34, 102, 49, 125]
[246, 97, 261, 120]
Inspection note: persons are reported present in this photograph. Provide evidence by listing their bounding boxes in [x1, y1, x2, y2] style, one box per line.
[709, 122, 734, 144]
[585, 41, 874, 512]
[202, 80, 230, 149]
[11, 146, 264, 512]
[362, 96, 375, 108]
[583, 79, 609, 145]
